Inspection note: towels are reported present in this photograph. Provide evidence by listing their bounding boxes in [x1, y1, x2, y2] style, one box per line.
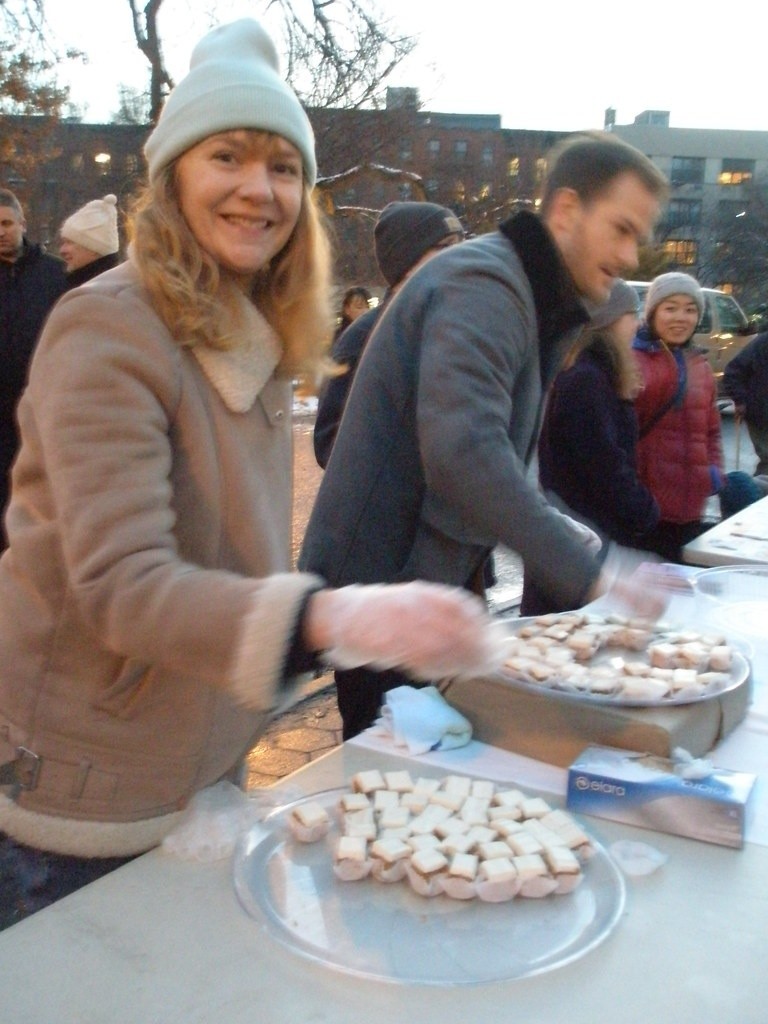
[374, 683, 476, 755]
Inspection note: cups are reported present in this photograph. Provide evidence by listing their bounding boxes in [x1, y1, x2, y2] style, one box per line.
[691, 564, 768, 636]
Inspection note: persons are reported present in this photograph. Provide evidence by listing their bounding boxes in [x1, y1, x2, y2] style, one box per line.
[308, 193, 469, 472]
[1, 19, 511, 931]
[722, 327, 768, 477]
[58, 193, 122, 295]
[623, 272, 729, 570]
[330, 286, 375, 349]
[516, 270, 663, 619]
[295, 132, 672, 743]
[0, 186, 68, 556]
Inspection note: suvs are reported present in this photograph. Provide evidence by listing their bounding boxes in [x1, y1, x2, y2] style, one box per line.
[617, 279, 768, 409]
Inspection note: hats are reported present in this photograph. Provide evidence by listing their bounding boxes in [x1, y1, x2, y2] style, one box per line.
[142, 17, 318, 191]
[644, 271, 705, 329]
[588, 277, 641, 329]
[60, 193, 121, 257]
[372, 199, 464, 286]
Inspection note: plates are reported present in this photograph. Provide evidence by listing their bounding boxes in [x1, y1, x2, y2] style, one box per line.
[483, 615, 751, 707]
[232, 783, 627, 989]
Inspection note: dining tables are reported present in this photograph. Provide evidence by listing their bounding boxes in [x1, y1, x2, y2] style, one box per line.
[4, 478, 768, 1020]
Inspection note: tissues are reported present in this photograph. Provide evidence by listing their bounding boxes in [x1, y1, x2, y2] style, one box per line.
[563, 737, 762, 855]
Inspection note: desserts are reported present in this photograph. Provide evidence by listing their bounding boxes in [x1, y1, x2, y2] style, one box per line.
[291, 610, 734, 902]
